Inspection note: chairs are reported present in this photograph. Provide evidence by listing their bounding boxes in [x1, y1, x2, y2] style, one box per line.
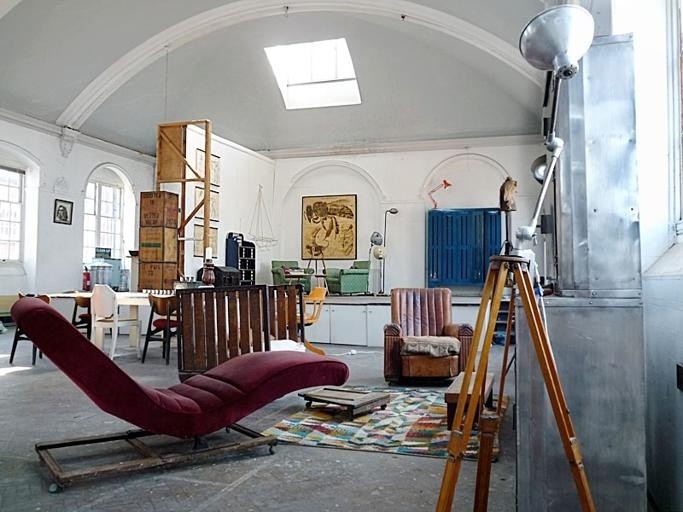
[325, 260, 371, 296]
[9, 283, 183, 366]
[10, 296, 350, 492]
[286, 287, 326, 355]
[382, 287, 476, 386]
[271, 260, 314, 295]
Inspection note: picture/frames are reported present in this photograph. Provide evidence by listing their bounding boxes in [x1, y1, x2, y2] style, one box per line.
[53, 198, 74, 225]
[300, 194, 357, 261]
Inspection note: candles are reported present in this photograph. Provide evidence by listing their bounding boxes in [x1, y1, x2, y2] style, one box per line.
[206, 246, 212, 259]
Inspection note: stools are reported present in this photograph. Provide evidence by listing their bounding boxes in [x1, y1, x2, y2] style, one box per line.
[445, 370, 496, 430]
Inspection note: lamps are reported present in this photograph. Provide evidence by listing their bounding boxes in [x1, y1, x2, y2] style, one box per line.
[502, 3, 595, 323]
[367, 208, 398, 297]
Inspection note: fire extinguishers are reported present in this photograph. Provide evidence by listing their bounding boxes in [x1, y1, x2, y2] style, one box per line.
[83, 266, 91, 291]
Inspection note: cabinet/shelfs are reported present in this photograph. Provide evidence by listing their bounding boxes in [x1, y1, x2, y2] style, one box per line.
[225, 232, 256, 285]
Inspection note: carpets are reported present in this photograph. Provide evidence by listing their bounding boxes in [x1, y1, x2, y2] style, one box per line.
[258, 384, 509, 463]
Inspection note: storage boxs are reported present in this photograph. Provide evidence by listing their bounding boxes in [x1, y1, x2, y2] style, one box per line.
[137, 191, 179, 289]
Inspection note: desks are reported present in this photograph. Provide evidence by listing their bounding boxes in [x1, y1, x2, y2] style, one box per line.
[285, 274, 329, 296]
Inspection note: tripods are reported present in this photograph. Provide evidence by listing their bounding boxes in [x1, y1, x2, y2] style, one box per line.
[434, 255, 596, 512]
[305, 260, 326, 273]
